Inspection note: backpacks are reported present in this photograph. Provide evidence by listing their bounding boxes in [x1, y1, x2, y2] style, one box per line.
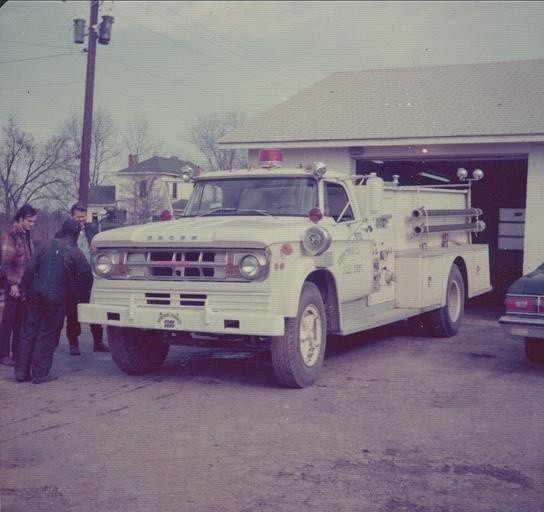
[64, 246, 92, 289]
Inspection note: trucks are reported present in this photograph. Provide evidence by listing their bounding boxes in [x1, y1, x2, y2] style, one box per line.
[77, 148, 492, 387]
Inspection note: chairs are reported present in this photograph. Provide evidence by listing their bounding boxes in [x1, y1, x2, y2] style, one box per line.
[235, 186, 317, 214]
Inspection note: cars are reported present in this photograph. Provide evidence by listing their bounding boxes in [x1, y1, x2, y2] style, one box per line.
[497, 261, 543, 359]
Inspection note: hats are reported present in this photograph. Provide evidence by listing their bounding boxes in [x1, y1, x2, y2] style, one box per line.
[63, 218, 81, 234]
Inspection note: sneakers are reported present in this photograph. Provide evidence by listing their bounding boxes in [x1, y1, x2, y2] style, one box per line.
[93, 344, 112, 352]
[0, 358, 15, 366]
[69, 345, 80, 356]
[17, 373, 57, 384]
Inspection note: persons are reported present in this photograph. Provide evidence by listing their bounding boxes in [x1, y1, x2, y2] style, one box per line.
[0, 203, 37, 366]
[53, 200, 111, 357]
[13, 217, 96, 386]
